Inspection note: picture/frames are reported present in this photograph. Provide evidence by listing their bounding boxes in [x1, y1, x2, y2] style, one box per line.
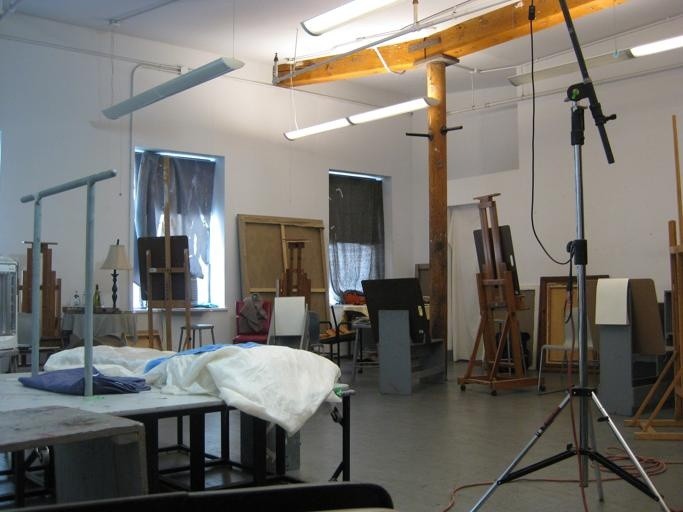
[536, 275, 611, 375]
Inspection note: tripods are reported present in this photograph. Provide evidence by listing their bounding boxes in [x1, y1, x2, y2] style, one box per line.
[470, 0, 672, 511]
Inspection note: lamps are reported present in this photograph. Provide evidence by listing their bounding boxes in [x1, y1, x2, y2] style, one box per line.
[285, 116, 350, 139]
[628, 27, 682, 64]
[99, 0, 244, 119]
[509, 49, 632, 88]
[101, 239, 132, 308]
[299, 2, 405, 36]
[348, 95, 438, 126]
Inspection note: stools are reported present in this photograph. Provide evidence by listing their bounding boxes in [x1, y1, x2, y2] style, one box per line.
[178, 324, 216, 353]
[350, 319, 379, 389]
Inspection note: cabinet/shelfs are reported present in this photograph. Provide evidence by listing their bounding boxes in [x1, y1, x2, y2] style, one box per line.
[379, 336, 444, 395]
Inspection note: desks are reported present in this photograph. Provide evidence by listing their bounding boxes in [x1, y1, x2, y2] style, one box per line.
[60, 311, 140, 343]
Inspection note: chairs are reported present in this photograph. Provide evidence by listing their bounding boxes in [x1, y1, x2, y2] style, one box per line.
[234, 299, 273, 343]
